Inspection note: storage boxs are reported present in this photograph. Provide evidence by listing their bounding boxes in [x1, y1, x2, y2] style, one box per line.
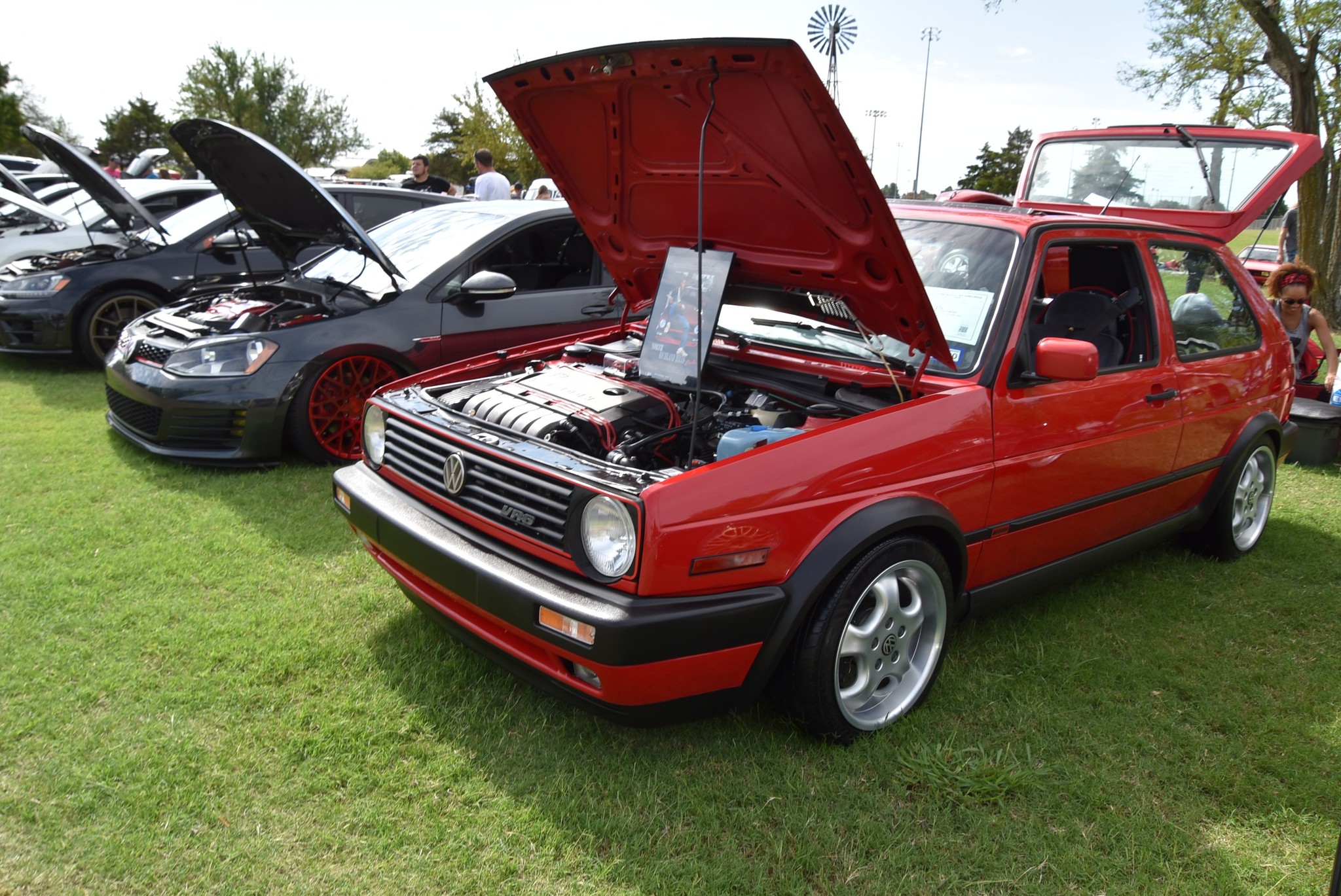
[1283, 420, 1341, 466]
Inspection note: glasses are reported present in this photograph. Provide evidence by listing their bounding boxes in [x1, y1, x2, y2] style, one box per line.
[1282, 297, 1307, 304]
[474, 150, 483, 163]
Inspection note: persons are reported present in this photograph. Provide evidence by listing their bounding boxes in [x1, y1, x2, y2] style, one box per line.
[1180, 196, 1215, 294]
[474, 148, 512, 201]
[401, 154, 457, 196]
[142, 166, 159, 179]
[1264, 258, 1338, 393]
[103, 154, 122, 179]
[511, 183, 527, 201]
[535, 185, 551, 200]
[1276, 206, 1298, 264]
[159, 170, 171, 179]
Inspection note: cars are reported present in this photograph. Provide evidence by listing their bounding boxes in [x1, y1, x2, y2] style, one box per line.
[103, 116, 672, 476]
[1236, 244, 1293, 286]
[303, 155, 570, 199]
[329, 39, 1325, 750]
[0, 142, 224, 270]
[0, 122, 476, 373]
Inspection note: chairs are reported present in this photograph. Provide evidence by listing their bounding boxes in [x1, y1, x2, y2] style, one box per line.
[507, 232, 546, 291]
[1030, 290, 1124, 375]
[554, 237, 592, 288]
[1295, 295, 1341, 400]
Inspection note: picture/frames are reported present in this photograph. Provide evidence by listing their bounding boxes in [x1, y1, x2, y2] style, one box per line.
[638, 246, 737, 385]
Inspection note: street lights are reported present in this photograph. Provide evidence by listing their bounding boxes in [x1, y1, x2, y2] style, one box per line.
[908, 26, 942, 200]
[865, 107, 888, 189]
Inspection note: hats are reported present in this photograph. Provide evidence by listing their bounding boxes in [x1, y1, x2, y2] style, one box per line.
[110, 155, 124, 165]
